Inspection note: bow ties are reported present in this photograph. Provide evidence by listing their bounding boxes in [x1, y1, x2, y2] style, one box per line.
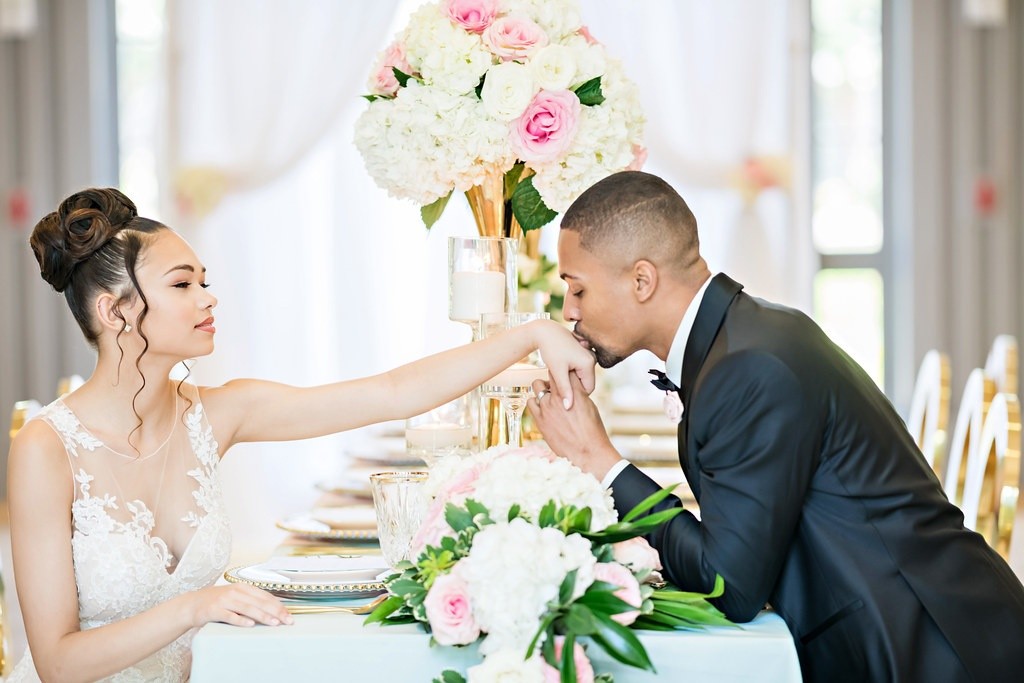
[648, 368, 679, 395]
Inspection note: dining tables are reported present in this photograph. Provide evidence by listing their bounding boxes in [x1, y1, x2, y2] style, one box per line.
[188, 461, 806, 683]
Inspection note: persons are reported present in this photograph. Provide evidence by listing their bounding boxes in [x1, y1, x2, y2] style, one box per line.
[526, 171, 1024, 683]
[6, 186, 597, 683]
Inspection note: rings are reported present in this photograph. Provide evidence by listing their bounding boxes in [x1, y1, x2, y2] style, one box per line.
[536, 390, 551, 404]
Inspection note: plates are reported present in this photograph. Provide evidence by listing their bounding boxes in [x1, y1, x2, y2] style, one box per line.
[224, 554, 401, 599]
[276, 568, 391, 582]
[275, 507, 384, 540]
[312, 513, 377, 529]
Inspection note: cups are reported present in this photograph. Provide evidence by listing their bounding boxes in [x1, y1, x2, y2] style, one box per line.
[368, 471, 431, 569]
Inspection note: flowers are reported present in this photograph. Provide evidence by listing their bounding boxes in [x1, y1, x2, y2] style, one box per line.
[351, 0, 656, 326]
[347, 442, 746, 682]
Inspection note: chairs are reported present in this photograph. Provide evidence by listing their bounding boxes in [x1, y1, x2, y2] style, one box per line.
[891, 331, 1024, 569]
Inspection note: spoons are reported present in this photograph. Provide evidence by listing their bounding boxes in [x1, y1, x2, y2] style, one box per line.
[284, 595, 388, 615]
